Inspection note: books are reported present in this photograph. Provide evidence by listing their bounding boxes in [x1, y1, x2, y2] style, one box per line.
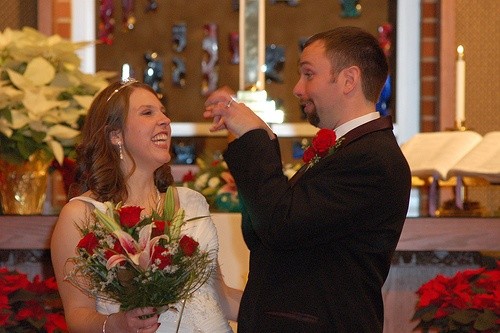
[399, 130, 500, 181]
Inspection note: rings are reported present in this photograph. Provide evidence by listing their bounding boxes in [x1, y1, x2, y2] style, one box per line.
[227, 99, 233, 108]
[136, 329, 140, 333]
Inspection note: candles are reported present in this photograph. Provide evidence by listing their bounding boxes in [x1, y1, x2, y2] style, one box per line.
[455, 46, 466, 117]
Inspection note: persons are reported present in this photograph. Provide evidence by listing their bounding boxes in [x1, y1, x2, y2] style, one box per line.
[203, 25, 412, 333]
[50, 79, 233, 333]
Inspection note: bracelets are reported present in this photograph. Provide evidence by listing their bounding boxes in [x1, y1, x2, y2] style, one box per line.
[102, 313, 113, 333]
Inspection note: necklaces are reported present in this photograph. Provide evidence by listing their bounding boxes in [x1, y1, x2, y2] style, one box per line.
[151, 187, 161, 220]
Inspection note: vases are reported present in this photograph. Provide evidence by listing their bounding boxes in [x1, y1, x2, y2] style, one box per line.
[0, 161, 48, 215]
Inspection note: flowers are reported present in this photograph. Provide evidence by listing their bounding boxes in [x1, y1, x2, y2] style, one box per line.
[302, 128, 336, 164]
[72, 205, 204, 303]
[418, 267, 500, 332]
[185, 158, 295, 210]
[0, 268, 69, 333]
[1, 28, 103, 160]
